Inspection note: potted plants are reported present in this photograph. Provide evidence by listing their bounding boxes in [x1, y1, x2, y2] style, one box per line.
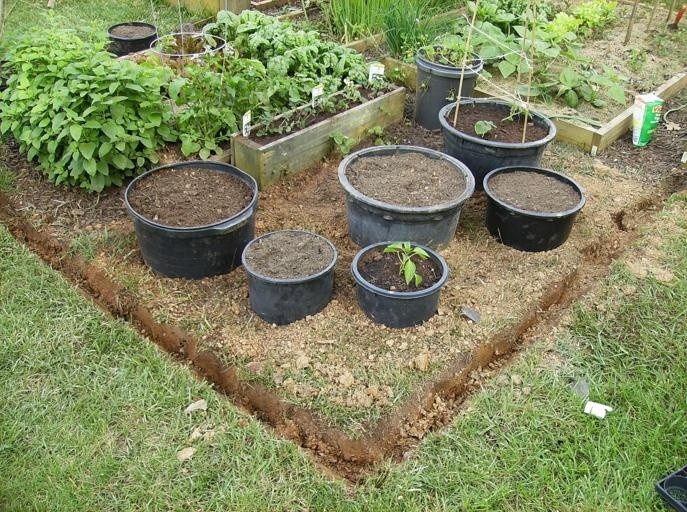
[349, 241, 448, 327]
[414, 33, 483, 132]
[438, 98, 556, 191]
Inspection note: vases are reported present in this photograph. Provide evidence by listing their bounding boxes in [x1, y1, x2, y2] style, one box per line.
[483, 166, 586, 252]
[241, 230, 337, 326]
[337, 145, 475, 252]
[124, 161, 258, 279]
[107, 22, 157, 59]
